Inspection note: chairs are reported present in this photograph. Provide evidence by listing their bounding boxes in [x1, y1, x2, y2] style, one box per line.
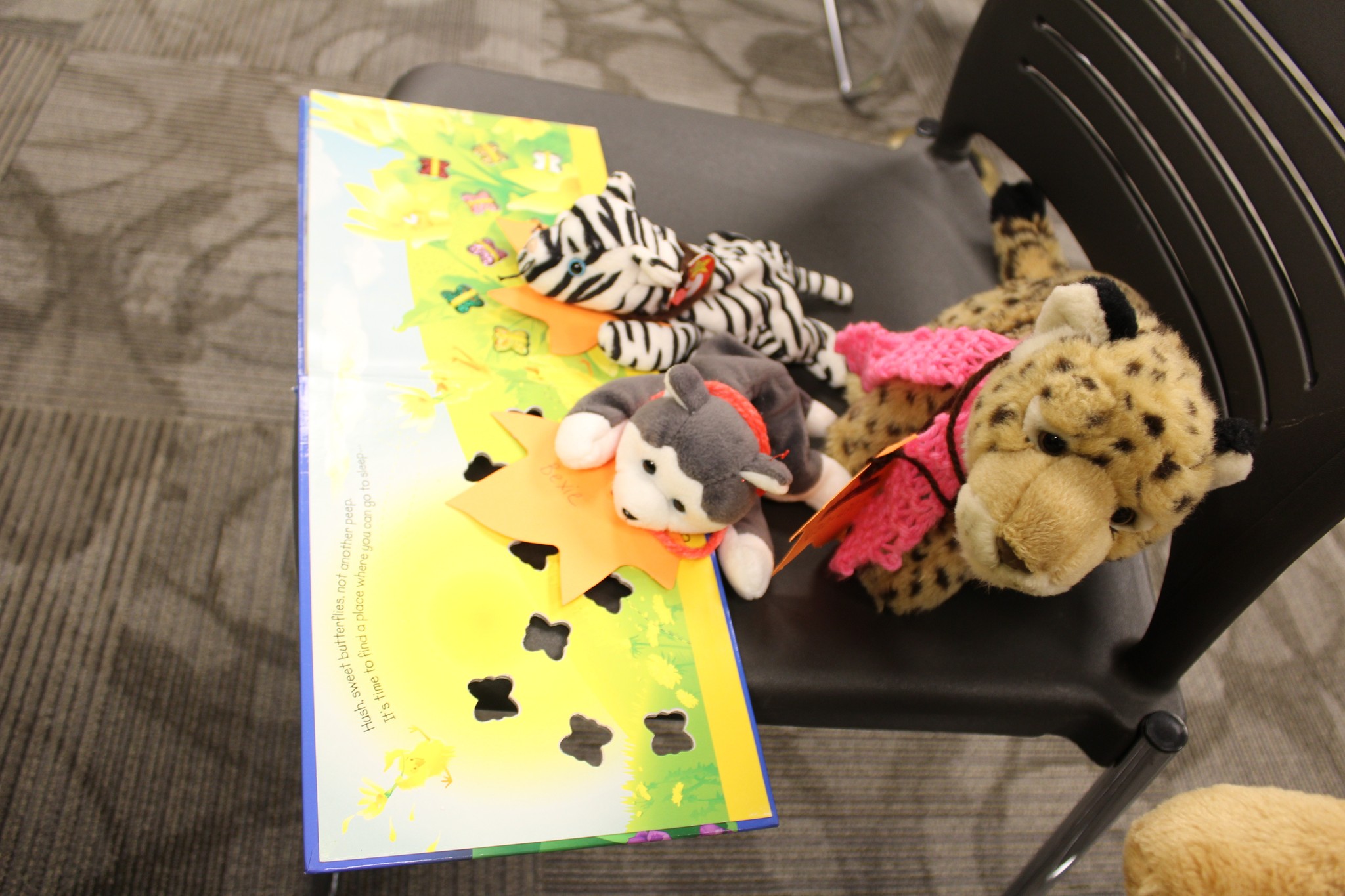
[290, 0, 1343, 895]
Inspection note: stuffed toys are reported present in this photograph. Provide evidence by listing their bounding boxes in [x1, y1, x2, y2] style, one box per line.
[552, 324, 854, 602]
[513, 168, 857, 392]
[762, 178, 1256, 617]
[1121, 783, 1345, 896]
